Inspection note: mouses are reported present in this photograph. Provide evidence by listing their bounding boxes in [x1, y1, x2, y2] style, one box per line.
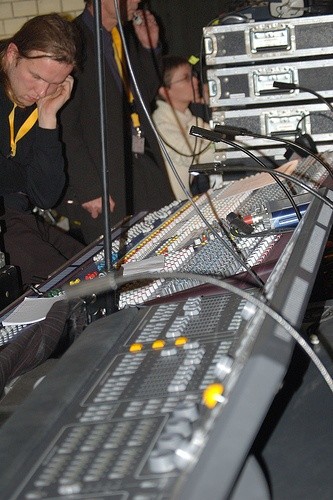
[218, 13, 246, 25]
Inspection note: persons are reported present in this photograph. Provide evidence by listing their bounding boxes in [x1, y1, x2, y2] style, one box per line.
[0, 12, 85, 284]
[58, 0, 160, 220]
[153, 57, 213, 201]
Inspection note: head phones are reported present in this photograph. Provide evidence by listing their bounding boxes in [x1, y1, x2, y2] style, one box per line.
[269, 0, 333, 19]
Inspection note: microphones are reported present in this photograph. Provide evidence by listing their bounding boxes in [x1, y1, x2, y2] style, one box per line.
[215, 125, 333, 177]
[64, 272, 332, 393]
[189, 162, 333, 209]
[189, 126, 302, 221]
[273, 81, 333, 112]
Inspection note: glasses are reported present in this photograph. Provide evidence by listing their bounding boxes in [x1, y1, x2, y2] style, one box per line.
[163, 71, 198, 89]
